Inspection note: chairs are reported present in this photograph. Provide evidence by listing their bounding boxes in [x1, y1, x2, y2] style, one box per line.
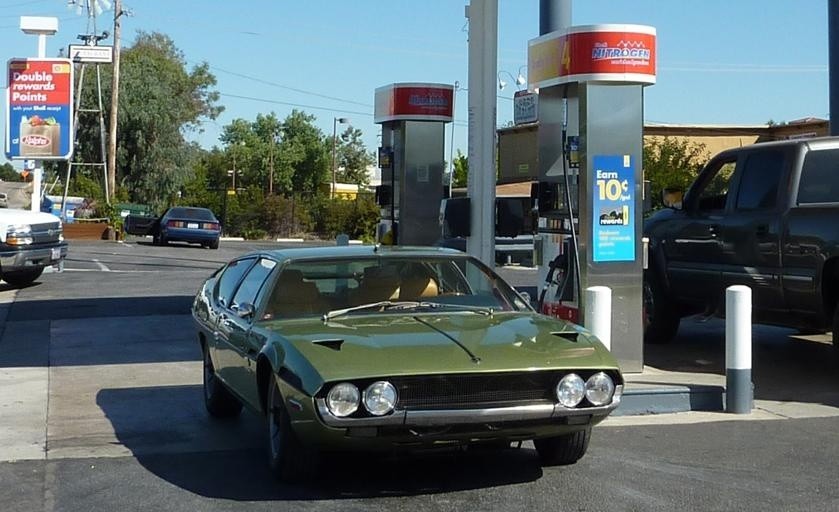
[394, 274, 442, 301]
[265, 280, 332, 319]
[346, 274, 404, 308]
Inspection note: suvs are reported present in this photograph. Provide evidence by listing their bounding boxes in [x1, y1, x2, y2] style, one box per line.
[437, 191, 536, 299]
[0, 207, 74, 289]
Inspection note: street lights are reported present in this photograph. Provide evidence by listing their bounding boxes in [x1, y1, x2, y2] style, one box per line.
[269, 130, 284, 192]
[18, 14, 60, 214]
[231, 138, 246, 190]
[331, 116, 350, 199]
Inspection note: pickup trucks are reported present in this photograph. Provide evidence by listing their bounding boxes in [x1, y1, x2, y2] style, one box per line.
[641, 132, 838, 359]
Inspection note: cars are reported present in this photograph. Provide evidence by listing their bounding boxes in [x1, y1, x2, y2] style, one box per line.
[122, 205, 222, 250]
[186, 226, 632, 487]
